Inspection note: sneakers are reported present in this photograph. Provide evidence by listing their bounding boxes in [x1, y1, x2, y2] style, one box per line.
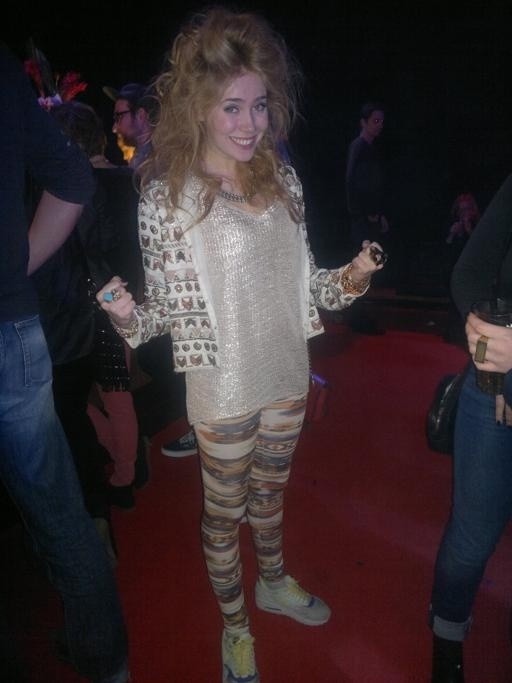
[432, 635, 466, 682]
[255, 576, 331, 626]
[134, 433, 150, 486]
[221, 627, 259, 682]
[162, 429, 198, 459]
[109, 484, 132, 508]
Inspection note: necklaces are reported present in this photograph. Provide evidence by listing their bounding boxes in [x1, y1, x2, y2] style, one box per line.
[216, 187, 254, 202]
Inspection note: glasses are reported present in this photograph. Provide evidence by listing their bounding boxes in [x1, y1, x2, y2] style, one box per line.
[113, 111, 131, 120]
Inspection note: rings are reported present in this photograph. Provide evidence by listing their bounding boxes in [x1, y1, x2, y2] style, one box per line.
[472, 335, 490, 363]
[370, 244, 388, 267]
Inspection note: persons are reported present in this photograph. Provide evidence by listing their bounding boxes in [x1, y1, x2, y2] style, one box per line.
[0, 0, 132, 683]
[25, 43, 198, 562]
[421, 169, 510, 681]
[438, 190, 480, 297]
[336, 102, 389, 337]
[90, 4, 391, 683]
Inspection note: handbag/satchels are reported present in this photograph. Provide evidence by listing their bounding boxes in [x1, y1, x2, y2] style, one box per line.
[91, 285, 130, 391]
[426, 373, 461, 453]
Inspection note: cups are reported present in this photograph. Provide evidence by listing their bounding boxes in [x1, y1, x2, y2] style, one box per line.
[470, 296, 512, 328]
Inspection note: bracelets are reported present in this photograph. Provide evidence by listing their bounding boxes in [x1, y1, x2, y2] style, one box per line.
[338, 264, 371, 297]
